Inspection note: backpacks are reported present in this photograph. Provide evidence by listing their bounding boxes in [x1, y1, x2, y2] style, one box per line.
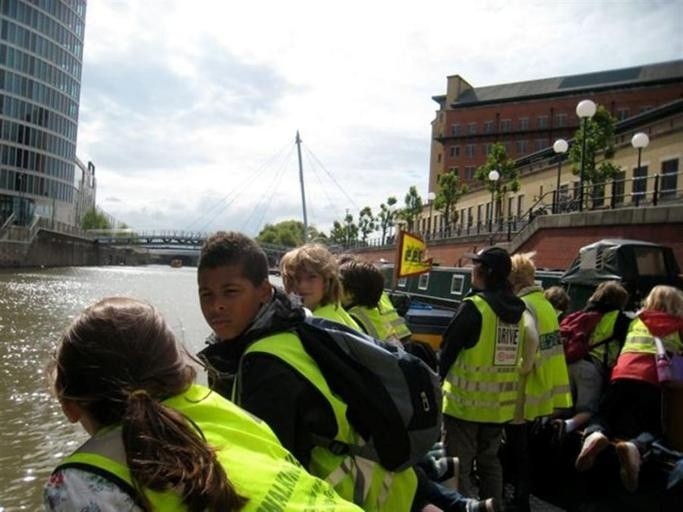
[292, 315, 442, 473]
[559, 309, 614, 364]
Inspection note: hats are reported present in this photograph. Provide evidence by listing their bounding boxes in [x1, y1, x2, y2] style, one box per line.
[465, 247, 512, 274]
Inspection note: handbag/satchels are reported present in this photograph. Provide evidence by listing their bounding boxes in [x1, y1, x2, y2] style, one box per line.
[656, 352, 683, 383]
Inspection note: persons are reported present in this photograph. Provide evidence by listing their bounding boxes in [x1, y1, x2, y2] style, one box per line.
[39, 296, 366, 511]
[278, 244, 497, 512]
[438, 247, 682, 512]
[192, 227, 443, 512]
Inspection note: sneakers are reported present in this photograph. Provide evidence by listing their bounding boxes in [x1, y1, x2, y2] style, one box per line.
[618, 442, 641, 491]
[532, 415, 567, 456]
[576, 433, 608, 470]
[478, 498, 499, 512]
[439, 456, 459, 483]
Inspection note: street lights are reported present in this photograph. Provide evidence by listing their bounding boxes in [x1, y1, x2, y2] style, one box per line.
[427, 192, 436, 240]
[552, 138, 568, 214]
[631, 132, 649, 206]
[488, 170, 499, 232]
[576, 99, 596, 211]
